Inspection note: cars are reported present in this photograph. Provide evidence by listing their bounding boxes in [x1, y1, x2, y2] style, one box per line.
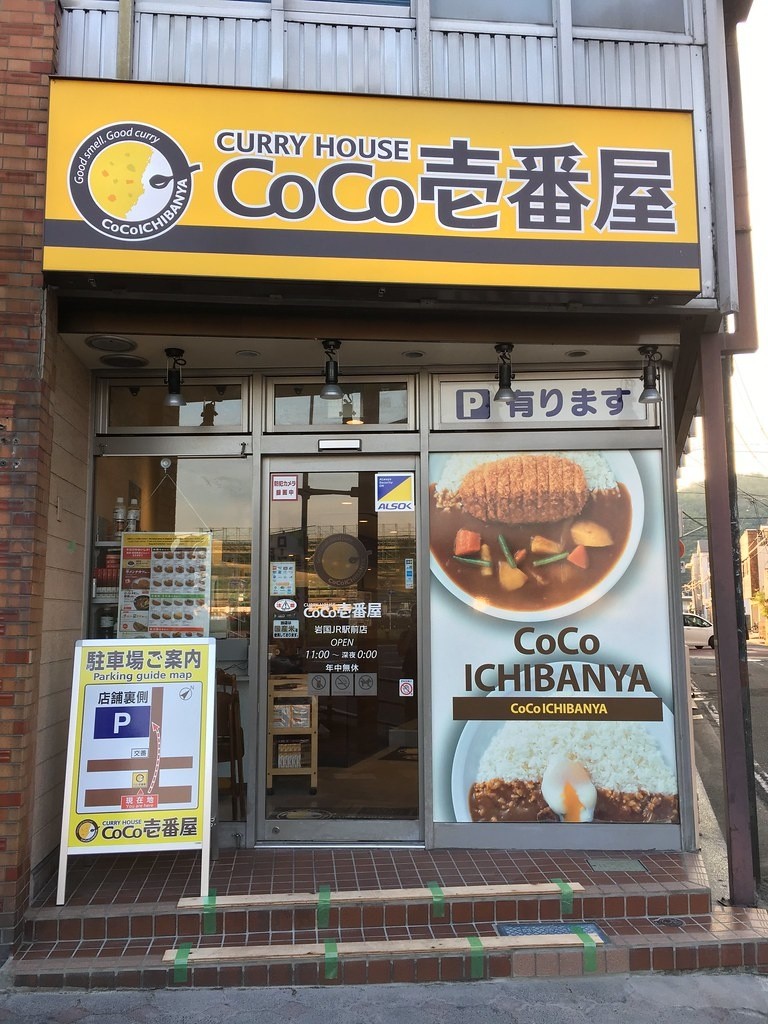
[682, 612, 715, 650]
[357, 614, 416, 642]
[396, 609, 410, 617]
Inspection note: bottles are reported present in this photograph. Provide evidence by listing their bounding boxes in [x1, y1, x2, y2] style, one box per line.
[126, 498, 141, 532]
[100, 607, 114, 639]
[113, 497, 126, 536]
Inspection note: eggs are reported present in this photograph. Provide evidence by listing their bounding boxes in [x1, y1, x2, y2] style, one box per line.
[542, 754, 597, 822]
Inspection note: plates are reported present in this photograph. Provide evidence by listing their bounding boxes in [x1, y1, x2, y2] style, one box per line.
[428, 450, 644, 623]
[451, 661, 680, 823]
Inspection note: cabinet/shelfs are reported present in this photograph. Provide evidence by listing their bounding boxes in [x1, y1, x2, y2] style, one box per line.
[268, 675, 319, 797]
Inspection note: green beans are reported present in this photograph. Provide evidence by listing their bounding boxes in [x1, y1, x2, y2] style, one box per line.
[453, 556, 491, 567]
[534, 552, 569, 566]
[499, 535, 517, 568]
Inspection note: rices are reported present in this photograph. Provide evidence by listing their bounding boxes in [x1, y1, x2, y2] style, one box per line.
[436, 453, 616, 498]
[476, 721, 677, 794]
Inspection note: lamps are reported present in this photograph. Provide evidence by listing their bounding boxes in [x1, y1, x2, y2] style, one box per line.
[163, 348, 188, 407]
[319, 341, 344, 400]
[339, 394, 357, 424]
[638, 347, 663, 403]
[200, 402, 219, 426]
[493, 343, 517, 402]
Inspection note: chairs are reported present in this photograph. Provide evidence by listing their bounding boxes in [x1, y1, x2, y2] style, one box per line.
[215, 667, 246, 822]
